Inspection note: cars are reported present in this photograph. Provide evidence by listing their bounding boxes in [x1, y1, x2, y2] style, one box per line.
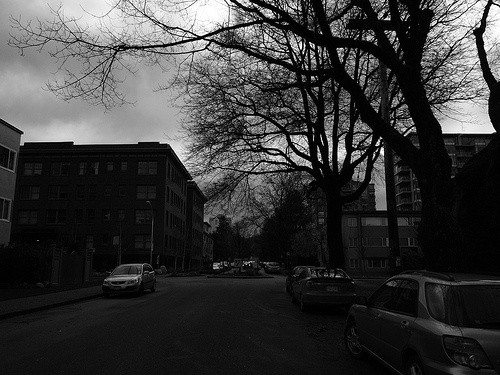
[285, 265, 316, 294]
[209, 257, 283, 277]
[101, 261, 158, 297]
[290, 265, 358, 313]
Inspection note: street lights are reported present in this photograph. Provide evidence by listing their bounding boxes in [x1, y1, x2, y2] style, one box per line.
[146, 200, 155, 265]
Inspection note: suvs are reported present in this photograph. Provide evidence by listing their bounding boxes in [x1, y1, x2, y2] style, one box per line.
[343, 267, 500, 375]
